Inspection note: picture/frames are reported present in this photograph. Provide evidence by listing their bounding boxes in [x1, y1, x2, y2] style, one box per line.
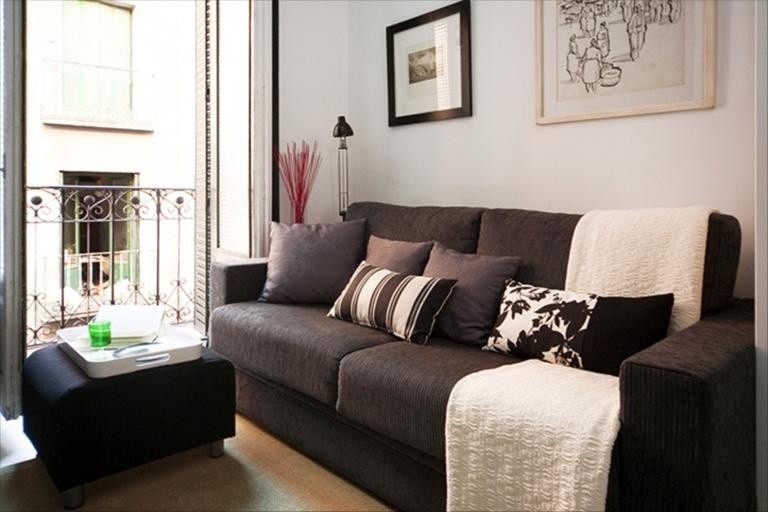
[533, 0, 719, 126]
[387, 0, 471, 127]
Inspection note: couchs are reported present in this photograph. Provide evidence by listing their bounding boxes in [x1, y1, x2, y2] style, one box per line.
[205, 201, 755, 512]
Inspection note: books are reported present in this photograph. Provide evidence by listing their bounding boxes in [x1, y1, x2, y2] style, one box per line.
[92, 304, 168, 345]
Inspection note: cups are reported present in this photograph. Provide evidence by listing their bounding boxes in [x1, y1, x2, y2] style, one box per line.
[87, 316, 113, 348]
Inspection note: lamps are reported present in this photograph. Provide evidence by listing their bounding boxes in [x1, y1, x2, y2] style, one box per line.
[332, 116, 354, 220]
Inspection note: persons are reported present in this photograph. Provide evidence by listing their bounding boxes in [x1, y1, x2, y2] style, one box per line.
[566, 0, 682, 91]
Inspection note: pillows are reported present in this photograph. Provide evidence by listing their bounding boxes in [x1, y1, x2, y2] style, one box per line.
[583, 292, 675, 379]
[366, 234, 434, 274]
[255, 219, 366, 303]
[327, 260, 458, 346]
[422, 240, 521, 348]
[480, 278, 600, 370]
